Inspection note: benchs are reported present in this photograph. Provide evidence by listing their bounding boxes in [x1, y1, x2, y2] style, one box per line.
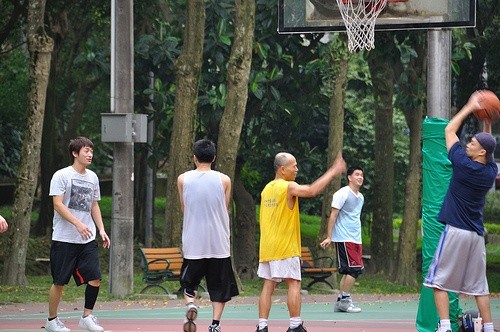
[301, 246, 338, 290]
[138, 247, 206, 295]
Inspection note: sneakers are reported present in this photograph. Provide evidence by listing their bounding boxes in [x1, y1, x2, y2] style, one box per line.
[207, 321, 224, 332]
[333, 291, 362, 314]
[181, 303, 199, 332]
[77, 313, 104, 331]
[282, 319, 313, 332]
[44, 317, 69, 332]
[256, 319, 272, 332]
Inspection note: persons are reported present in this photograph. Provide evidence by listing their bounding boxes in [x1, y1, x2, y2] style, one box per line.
[256, 151, 346, 332]
[422, 90, 498, 332]
[44, 136, 111, 332]
[176, 139, 239, 332]
[0, 216, 9, 233]
[320, 166, 365, 313]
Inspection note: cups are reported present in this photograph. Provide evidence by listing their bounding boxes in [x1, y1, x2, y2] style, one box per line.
[472, 317, 482, 332]
[462, 314, 472, 331]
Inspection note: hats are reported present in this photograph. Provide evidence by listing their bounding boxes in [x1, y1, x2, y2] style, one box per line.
[474, 132, 500, 165]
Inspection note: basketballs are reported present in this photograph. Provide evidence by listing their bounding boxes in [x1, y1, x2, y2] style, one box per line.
[473, 90, 500, 122]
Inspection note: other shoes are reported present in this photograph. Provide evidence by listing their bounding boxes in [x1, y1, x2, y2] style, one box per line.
[433, 319, 455, 332]
